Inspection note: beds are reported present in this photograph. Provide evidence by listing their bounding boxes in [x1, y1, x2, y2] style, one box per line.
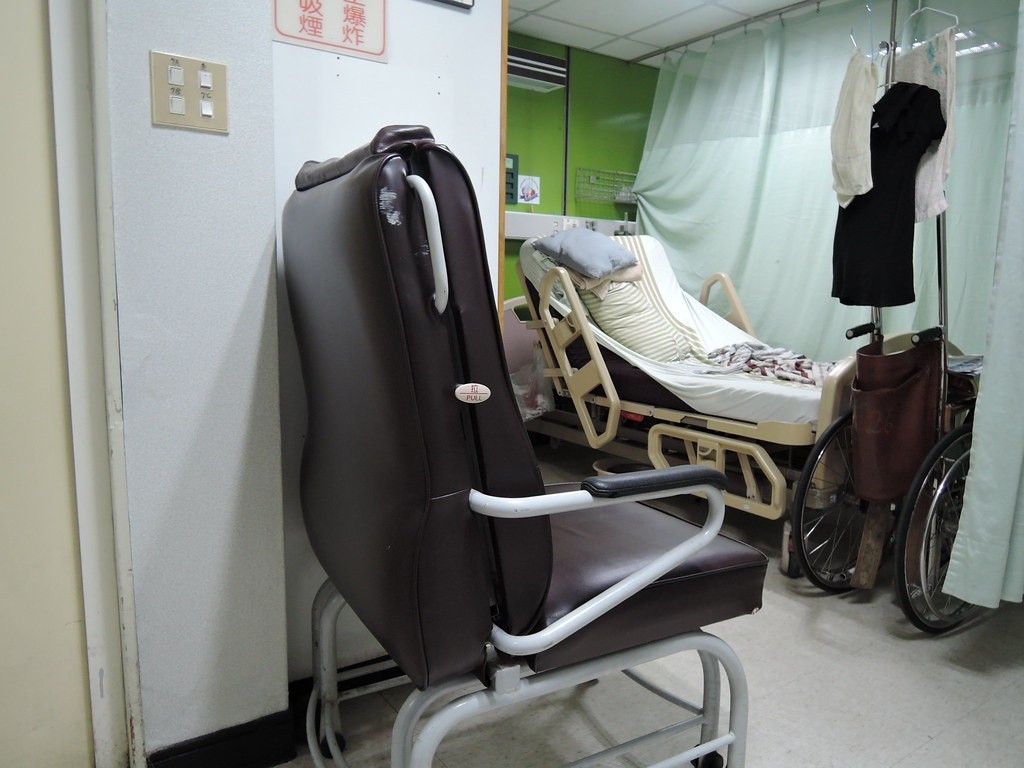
[515, 235, 965, 520]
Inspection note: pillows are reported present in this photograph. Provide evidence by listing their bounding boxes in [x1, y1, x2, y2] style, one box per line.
[529, 226, 638, 277]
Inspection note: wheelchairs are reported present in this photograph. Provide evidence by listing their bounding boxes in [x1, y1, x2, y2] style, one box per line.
[792, 322, 986, 633]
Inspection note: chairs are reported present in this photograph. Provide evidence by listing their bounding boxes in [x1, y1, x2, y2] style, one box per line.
[282, 126, 768, 768]
